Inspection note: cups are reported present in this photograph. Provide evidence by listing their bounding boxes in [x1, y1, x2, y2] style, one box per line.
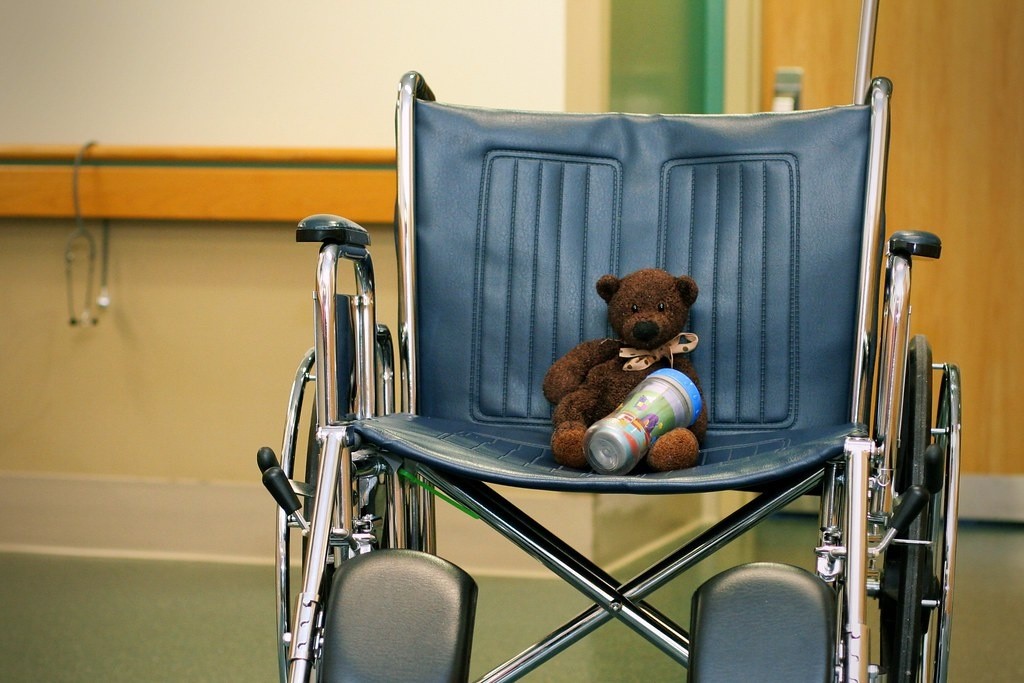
[581, 367, 702, 475]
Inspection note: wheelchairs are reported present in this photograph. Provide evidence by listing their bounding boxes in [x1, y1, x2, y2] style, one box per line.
[257, 71, 964, 683]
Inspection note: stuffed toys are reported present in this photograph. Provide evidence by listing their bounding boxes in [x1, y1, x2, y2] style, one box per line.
[543, 269, 706, 471]
